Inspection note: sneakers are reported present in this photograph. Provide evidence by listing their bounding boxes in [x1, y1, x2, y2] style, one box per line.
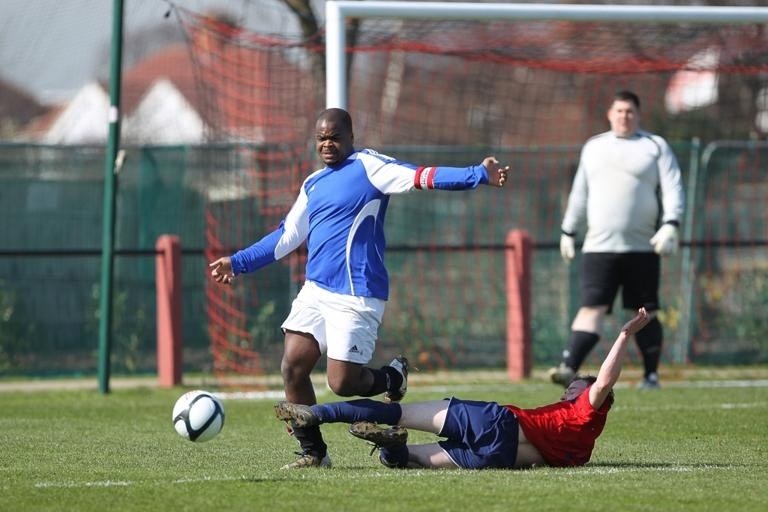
[273, 400, 314, 427]
[385, 357, 409, 402]
[643, 371, 659, 391]
[551, 367, 576, 386]
[348, 422, 408, 449]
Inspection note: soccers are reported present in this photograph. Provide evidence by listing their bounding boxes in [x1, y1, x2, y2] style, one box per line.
[172, 390, 224, 442]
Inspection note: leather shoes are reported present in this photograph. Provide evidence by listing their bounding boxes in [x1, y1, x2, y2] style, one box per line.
[280, 452, 332, 471]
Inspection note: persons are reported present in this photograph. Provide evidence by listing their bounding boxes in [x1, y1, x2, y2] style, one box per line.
[551, 91, 686, 390]
[274, 306, 651, 468]
[208, 109, 510, 468]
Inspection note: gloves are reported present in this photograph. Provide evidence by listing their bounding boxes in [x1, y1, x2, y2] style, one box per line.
[560, 233, 575, 265]
[650, 222, 680, 257]
